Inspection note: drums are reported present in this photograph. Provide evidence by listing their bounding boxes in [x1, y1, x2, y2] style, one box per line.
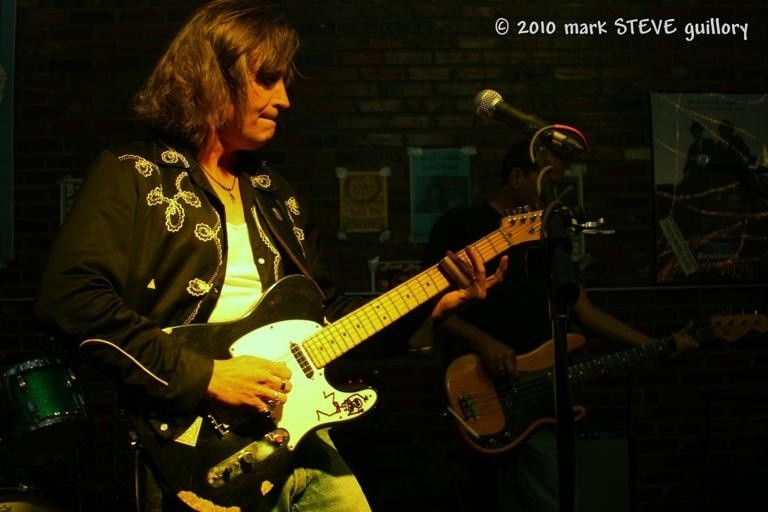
[1, 360, 89, 438]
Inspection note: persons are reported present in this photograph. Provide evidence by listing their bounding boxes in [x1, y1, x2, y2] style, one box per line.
[420, 137, 699, 512]
[32, 0, 508, 512]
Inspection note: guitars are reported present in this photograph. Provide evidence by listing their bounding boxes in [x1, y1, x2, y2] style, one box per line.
[445, 309, 766, 454]
[137, 205, 547, 512]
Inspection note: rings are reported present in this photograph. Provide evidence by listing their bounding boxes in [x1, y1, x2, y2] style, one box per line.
[280, 379, 285, 391]
[269, 391, 281, 409]
[262, 407, 273, 418]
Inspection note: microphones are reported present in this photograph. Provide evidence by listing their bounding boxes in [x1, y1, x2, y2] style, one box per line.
[474, 88, 586, 155]
